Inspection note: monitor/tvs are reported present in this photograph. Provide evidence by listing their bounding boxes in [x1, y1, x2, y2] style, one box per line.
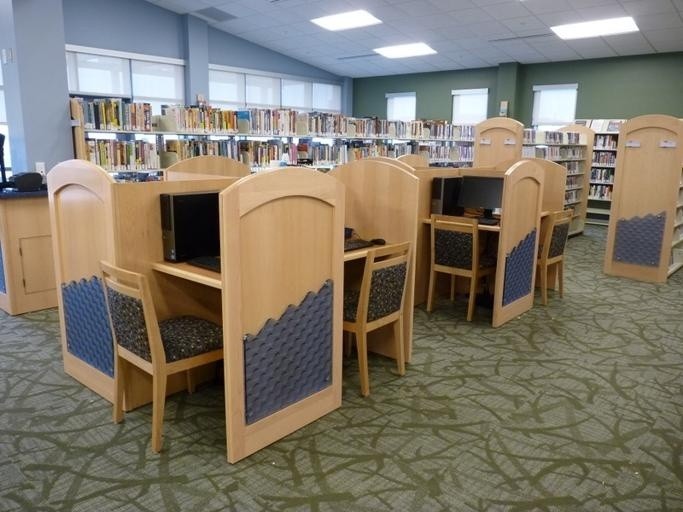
[458, 176, 504, 218]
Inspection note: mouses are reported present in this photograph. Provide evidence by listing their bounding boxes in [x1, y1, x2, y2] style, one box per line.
[372, 239, 385, 244]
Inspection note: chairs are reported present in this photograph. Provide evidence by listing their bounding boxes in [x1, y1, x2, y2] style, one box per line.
[96, 261, 225, 453]
[536, 209, 572, 306]
[424, 211, 493, 322]
[343, 239, 414, 399]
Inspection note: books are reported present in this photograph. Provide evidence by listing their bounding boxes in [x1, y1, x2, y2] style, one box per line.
[587, 134, 616, 198]
[522, 129, 583, 214]
[80, 94, 477, 168]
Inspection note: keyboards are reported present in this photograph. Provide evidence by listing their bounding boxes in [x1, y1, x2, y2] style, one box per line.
[473, 217, 500, 225]
[344, 239, 373, 252]
[187, 256, 220, 273]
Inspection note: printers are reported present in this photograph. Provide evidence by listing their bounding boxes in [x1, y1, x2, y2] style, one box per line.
[2, 172, 42, 192]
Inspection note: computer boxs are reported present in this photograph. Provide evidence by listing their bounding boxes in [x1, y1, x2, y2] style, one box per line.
[160, 191, 220, 263]
[431, 176, 464, 216]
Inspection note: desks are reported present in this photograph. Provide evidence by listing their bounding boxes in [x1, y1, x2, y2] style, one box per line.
[423, 213, 502, 231]
[344, 239, 400, 262]
[155, 258, 223, 292]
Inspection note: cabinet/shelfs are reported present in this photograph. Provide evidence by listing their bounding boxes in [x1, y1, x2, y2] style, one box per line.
[585, 133, 618, 227]
[67, 96, 474, 179]
[471, 118, 590, 235]
[0, 189, 58, 316]
[604, 115, 683, 283]
